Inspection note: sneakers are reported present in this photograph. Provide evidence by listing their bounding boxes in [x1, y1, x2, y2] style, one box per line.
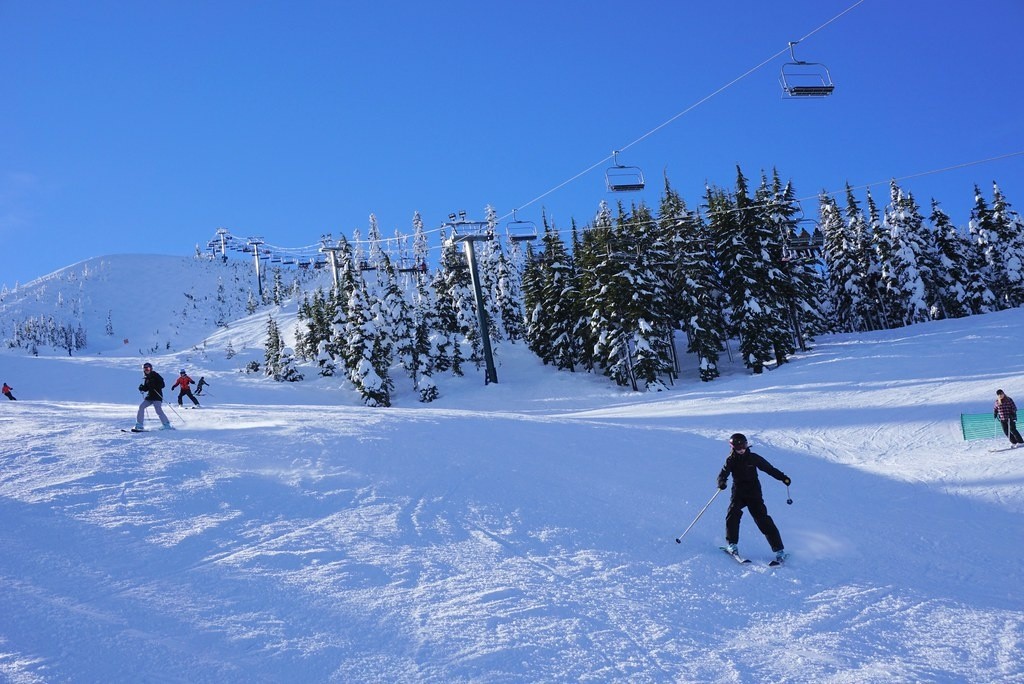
[133, 423, 143, 429]
[1010, 442, 1024, 449]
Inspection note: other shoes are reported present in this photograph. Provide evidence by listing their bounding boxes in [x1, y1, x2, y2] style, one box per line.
[775, 549, 784, 558]
[140, 425, 141, 426]
[727, 543, 738, 552]
[196, 404, 200, 407]
[179, 404, 182, 407]
[162, 422, 170, 429]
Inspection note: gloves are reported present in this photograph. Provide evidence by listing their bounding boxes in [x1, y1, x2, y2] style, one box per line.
[717, 481, 727, 490]
[784, 477, 793, 486]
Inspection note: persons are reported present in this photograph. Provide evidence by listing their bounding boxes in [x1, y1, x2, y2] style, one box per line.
[2, 382, 17, 401]
[172, 369, 201, 407]
[993, 389, 1024, 448]
[131, 362, 175, 432]
[193, 375, 212, 396]
[718, 433, 794, 562]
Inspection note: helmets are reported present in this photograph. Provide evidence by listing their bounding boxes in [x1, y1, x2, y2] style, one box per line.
[179, 369, 185, 372]
[729, 433, 748, 450]
[142, 363, 152, 370]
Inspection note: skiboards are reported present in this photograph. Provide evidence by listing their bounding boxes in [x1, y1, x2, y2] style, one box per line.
[987, 445, 1024, 452]
[120, 427, 175, 432]
[192, 393, 206, 396]
[177, 406, 203, 409]
[718, 545, 791, 566]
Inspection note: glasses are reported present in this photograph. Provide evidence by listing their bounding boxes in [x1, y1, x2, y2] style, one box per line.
[144, 368, 151, 371]
[180, 372, 185, 374]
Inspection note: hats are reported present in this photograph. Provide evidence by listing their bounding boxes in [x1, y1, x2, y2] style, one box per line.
[997, 390, 1003, 395]
